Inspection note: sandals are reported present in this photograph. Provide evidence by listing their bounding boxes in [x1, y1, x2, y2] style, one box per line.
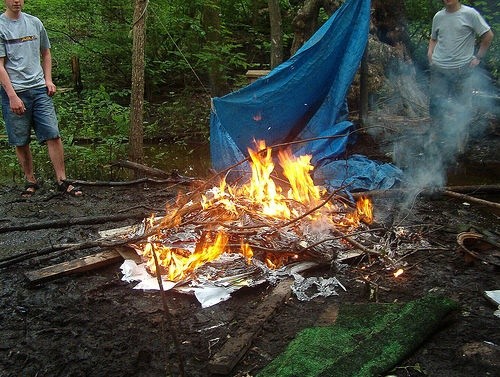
[21, 181, 39, 198]
[58, 181, 83, 197]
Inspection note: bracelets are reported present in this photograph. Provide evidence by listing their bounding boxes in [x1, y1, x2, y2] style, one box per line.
[475, 52, 483, 59]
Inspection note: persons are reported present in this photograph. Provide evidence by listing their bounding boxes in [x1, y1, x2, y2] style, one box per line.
[0, 0, 83, 197]
[424, 0, 494, 154]
